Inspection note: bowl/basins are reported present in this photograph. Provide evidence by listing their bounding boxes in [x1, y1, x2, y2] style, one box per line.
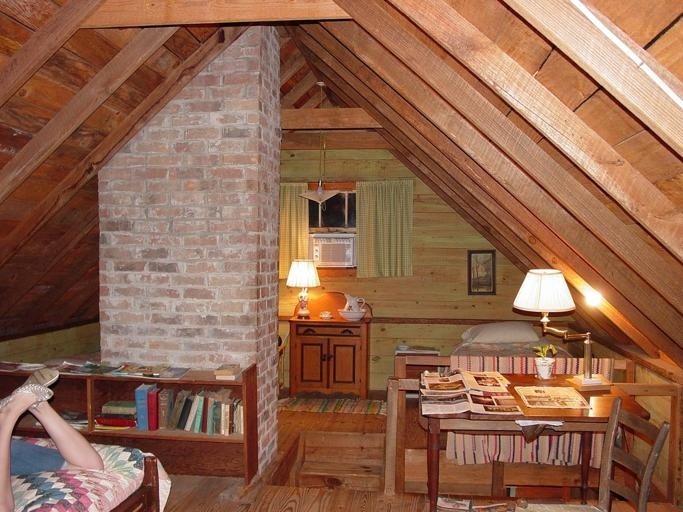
[337, 309, 367, 322]
[398, 345, 409, 351]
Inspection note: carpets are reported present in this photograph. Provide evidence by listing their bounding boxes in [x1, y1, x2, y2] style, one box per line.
[277, 396, 389, 418]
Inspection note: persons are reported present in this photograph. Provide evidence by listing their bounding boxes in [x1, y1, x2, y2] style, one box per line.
[0, 366, 103, 512]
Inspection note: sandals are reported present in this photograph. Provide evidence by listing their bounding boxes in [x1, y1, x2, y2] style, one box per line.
[0, 368, 59, 410]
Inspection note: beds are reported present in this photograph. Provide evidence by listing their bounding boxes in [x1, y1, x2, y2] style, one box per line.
[445, 341, 575, 373]
[10, 434, 172, 512]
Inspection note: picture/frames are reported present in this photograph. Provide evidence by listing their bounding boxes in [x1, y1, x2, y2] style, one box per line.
[467, 248, 497, 295]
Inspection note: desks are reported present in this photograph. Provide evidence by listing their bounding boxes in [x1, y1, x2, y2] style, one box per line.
[417, 372, 651, 512]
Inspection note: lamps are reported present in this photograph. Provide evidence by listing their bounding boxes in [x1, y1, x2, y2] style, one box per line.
[299, 81, 340, 206]
[512, 268, 613, 390]
[285, 259, 321, 318]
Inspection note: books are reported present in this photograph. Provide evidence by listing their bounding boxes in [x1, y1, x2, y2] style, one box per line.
[0, 361, 245, 381]
[33, 411, 88, 430]
[411, 368, 591, 414]
[95, 384, 243, 438]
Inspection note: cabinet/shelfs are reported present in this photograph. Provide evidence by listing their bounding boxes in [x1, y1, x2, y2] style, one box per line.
[288, 292, 373, 398]
[0, 362, 259, 485]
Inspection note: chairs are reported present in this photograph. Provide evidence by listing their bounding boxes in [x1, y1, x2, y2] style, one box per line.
[514, 396, 670, 512]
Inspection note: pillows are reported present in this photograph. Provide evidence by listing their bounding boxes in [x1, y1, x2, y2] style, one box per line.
[461, 320, 540, 347]
